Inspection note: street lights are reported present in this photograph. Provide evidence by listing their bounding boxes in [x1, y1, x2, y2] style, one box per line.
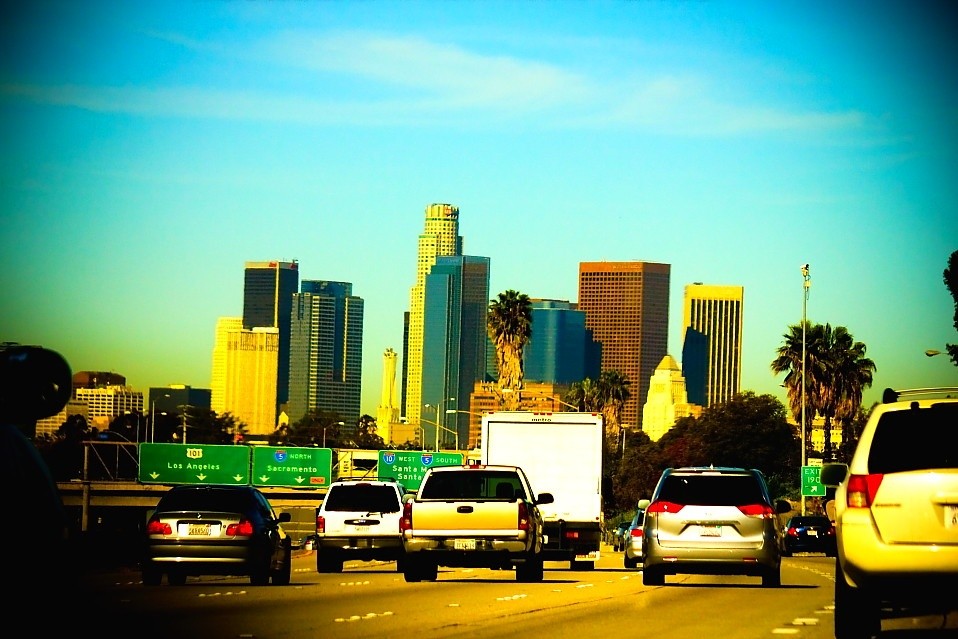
[404, 421, 424, 452]
[424, 398, 456, 452]
[399, 416, 459, 452]
[124, 394, 170, 480]
[323, 422, 345, 448]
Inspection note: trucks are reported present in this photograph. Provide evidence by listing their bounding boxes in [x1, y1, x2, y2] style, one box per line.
[482, 410, 603, 570]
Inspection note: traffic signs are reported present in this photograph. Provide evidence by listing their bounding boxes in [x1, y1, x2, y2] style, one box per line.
[252, 445, 332, 489]
[801, 465, 826, 496]
[139, 443, 250, 486]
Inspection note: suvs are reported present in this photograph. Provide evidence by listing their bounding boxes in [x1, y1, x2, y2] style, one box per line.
[779, 515, 834, 556]
[820, 388, 958, 638]
[315, 477, 409, 573]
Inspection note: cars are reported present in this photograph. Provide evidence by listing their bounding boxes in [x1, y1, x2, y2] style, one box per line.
[146, 486, 292, 587]
[0, 345, 80, 638]
[298, 536, 316, 550]
[613, 467, 791, 588]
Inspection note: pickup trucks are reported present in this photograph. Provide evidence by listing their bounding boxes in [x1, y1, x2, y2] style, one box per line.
[402, 465, 554, 582]
